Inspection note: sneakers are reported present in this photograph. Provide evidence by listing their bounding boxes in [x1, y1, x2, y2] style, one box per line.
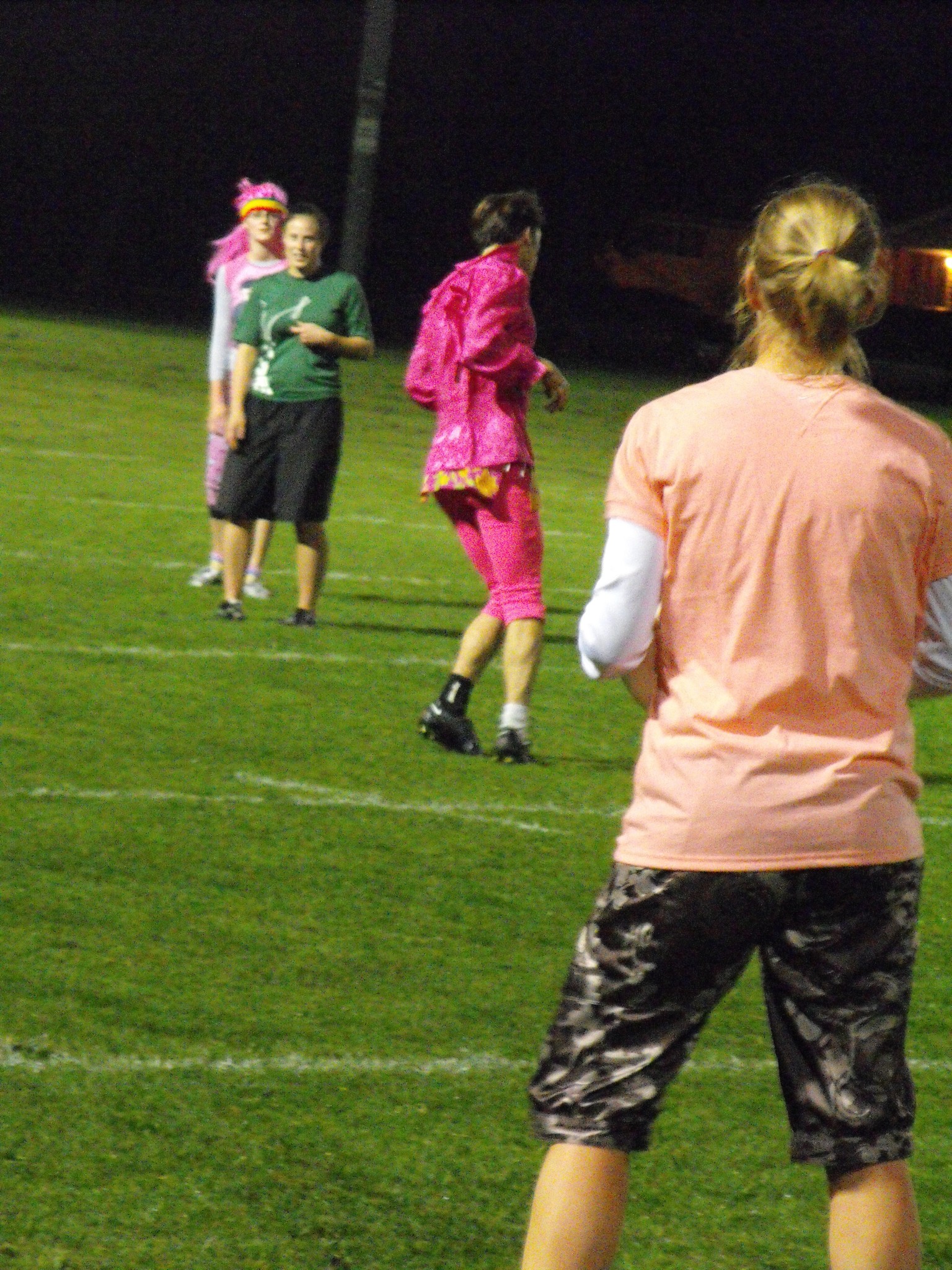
[496, 729, 540, 765]
[244, 577, 271, 601]
[288, 609, 315, 628]
[188, 565, 220, 589]
[417, 704, 488, 758]
[216, 601, 247, 624]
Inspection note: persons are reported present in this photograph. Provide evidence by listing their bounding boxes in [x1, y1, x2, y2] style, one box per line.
[399, 190, 572, 769]
[188, 179, 373, 628]
[515, 183, 951, 1269]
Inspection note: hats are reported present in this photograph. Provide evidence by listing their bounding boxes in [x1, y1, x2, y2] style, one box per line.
[232, 177, 289, 222]
